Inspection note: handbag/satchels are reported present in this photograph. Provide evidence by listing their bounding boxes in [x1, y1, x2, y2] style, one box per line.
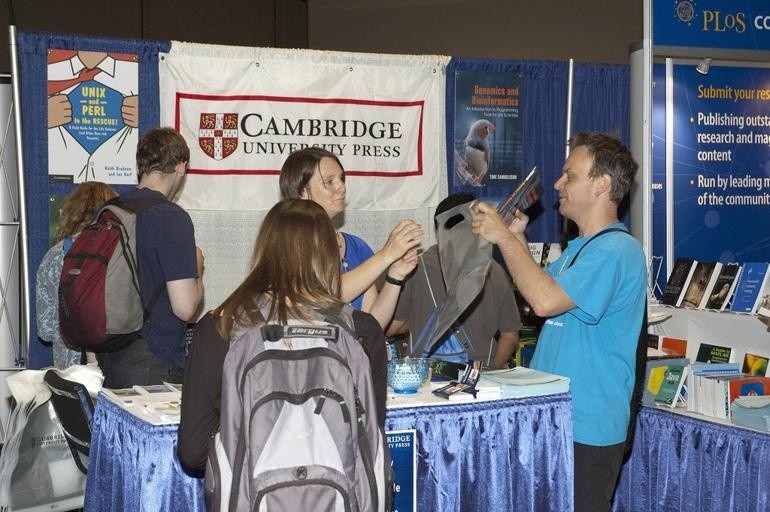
[423, 201, 497, 359]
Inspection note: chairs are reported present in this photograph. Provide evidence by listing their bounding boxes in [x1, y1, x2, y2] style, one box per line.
[43, 369, 95, 476]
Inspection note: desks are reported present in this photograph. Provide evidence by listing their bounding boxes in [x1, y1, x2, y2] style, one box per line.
[98, 378, 572, 511]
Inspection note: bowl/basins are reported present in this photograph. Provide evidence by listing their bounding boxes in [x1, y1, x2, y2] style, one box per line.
[384, 357, 429, 396]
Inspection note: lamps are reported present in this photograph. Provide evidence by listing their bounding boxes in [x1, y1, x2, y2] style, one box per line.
[696, 58, 713, 75]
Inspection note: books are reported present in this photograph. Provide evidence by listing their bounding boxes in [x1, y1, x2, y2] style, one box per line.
[102, 378, 184, 425]
[431, 376, 501, 406]
[640, 254, 769, 436]
[496, 166, 547, 226]
[478, 364, 561, 386]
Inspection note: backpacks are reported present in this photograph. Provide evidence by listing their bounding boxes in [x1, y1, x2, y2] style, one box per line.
[57, 196, 164, 355]
[203, 292, 396, 512]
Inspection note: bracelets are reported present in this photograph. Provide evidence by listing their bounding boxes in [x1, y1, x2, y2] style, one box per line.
[383, 272, 408, 288]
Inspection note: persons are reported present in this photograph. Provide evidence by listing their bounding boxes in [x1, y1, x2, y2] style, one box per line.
[46, 47, 141, 190]
[176, 198, 390, 512]
[467, 129, 652, 511]
[32, 178, 123, 368]
[384, 191, 524, 375]
[276, 143, 424, 337]
[93, 124, 206, 389]
